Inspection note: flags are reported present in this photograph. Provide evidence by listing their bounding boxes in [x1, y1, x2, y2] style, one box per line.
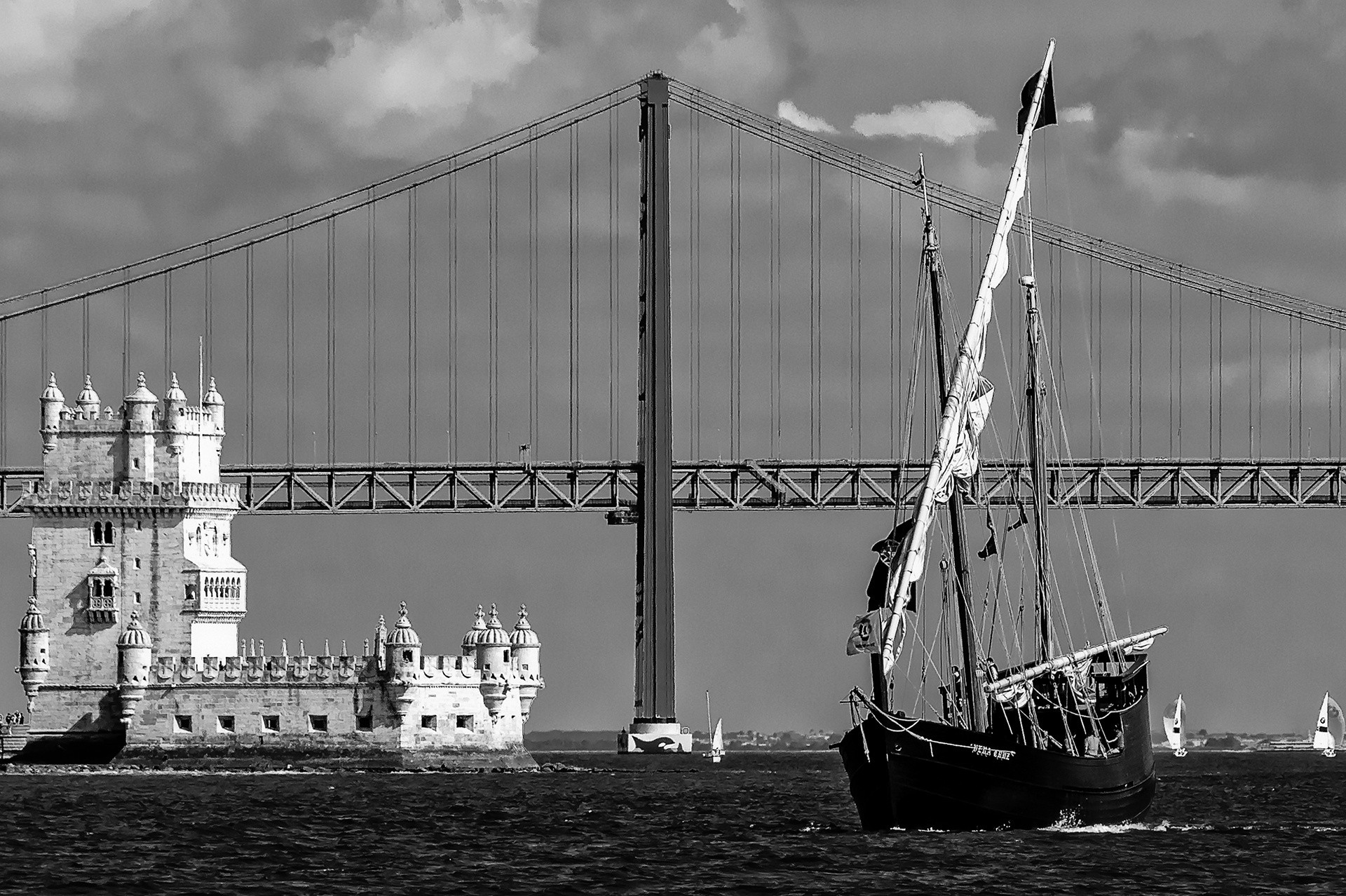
[865, 518, 917, 614]
[845, 608, 883, 657]
[1017, 59, 1058, 136]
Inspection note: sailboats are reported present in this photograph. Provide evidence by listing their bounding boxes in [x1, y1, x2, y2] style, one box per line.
[1161, 692, 1190, 758]
[704, 689, 727, 763]
[1311, 690, 1346, 758]
[828, 31, 1173, 831]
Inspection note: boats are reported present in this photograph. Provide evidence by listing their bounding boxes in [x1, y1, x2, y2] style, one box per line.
[1256, 739, 1317, 751]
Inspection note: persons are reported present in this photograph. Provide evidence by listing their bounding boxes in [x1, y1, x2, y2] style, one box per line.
[985, 657, 1001, 695]
[1061, 722, 1126, 760]
[951, 665, 966, 702]
